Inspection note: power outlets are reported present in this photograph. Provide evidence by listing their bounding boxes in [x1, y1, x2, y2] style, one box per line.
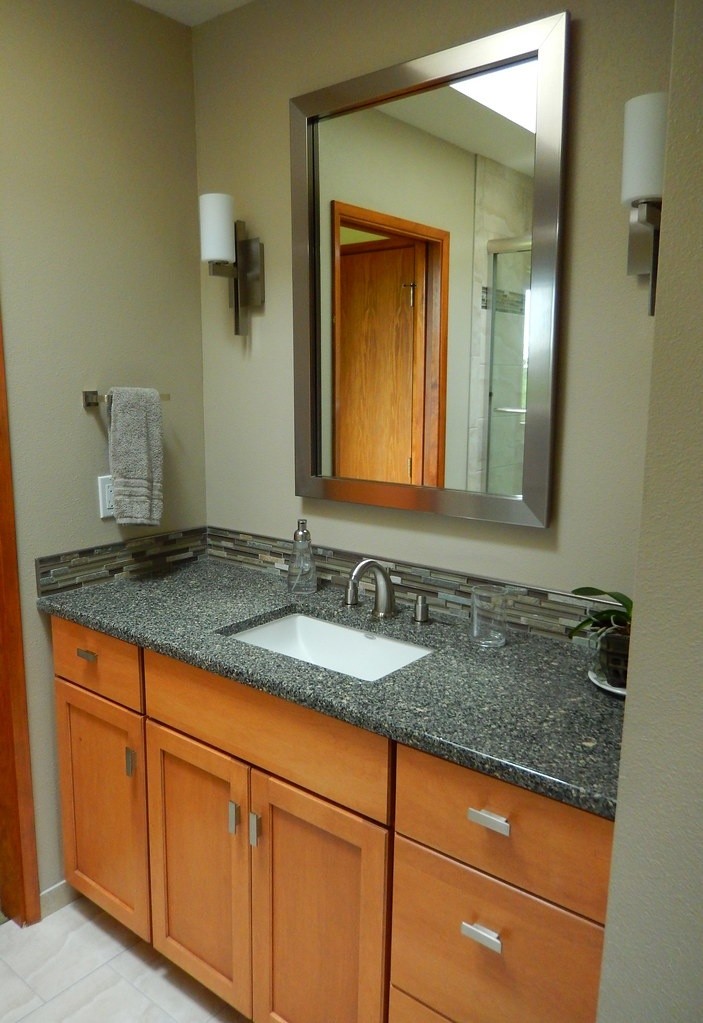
[98, 475, 114, 519]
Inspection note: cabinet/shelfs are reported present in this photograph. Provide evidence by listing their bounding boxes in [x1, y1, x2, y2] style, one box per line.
[51, 614, 151, 945]
[387, 740, 615, 1023]
[142, 647, 394, 1023]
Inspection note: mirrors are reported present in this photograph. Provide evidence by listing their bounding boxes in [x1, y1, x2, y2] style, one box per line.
[287, 10, 566, 529]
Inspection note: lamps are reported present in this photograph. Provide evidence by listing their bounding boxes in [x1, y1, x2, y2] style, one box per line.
[198, 193, 265, 336]
[620, 90, 669, 316]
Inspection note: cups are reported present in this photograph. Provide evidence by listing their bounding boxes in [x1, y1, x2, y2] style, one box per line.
[471, 585, 507, 648]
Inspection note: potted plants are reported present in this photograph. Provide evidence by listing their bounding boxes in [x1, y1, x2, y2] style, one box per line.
[567, 586, 633, 697]
